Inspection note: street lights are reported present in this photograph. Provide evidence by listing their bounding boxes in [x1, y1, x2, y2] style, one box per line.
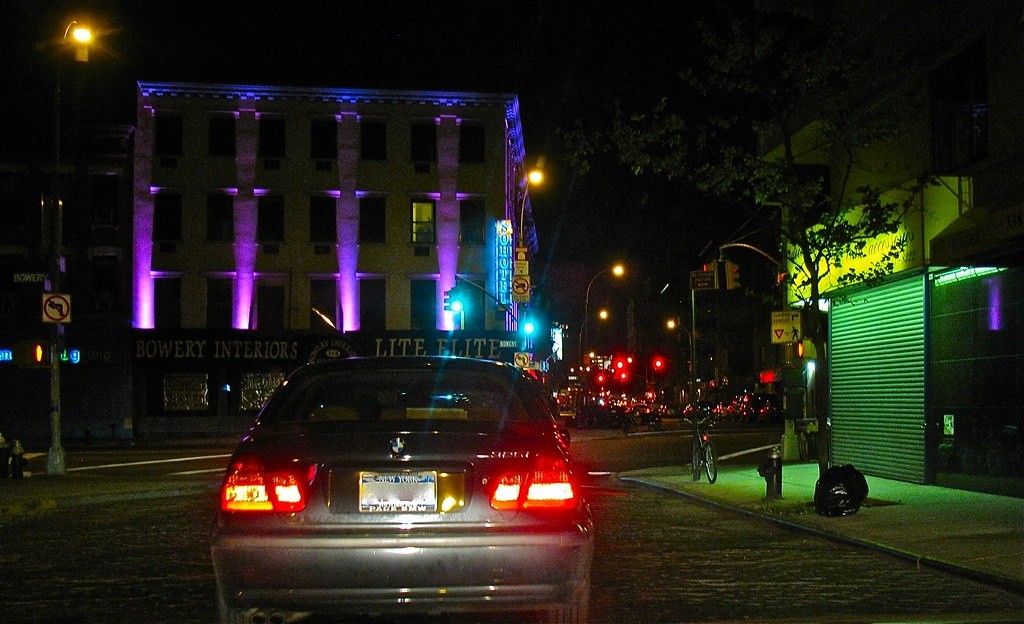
[666, 319, 694, 400]
[43, 19, 97, 476]
[584, 264, 625, 349]
[516, 167, 546, 319]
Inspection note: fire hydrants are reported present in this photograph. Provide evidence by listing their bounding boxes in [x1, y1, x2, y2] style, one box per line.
[759, 444, 779, 497]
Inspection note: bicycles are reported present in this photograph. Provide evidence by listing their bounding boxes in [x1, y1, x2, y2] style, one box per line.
[684, 414, 718, 485]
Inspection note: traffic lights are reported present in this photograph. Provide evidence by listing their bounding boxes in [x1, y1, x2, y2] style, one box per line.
[702, 260, 720, 291]
[33, 340, 52, 369]
[443, 286, 457, 311]
[724, 260, 740, 290]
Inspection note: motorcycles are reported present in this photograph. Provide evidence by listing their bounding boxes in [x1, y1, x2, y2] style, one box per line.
[621, 404, 662, 434]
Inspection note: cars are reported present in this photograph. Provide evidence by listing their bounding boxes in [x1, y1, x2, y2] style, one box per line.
[207, 352, 594, 623]
